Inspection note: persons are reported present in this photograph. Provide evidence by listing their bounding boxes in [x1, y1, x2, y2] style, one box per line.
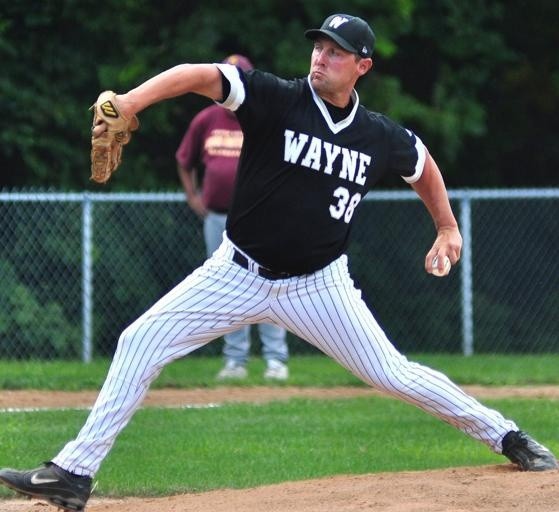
[0, 13, 557, 511]
[173, 51, 296, 382]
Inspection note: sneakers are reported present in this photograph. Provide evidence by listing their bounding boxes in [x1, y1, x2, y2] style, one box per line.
[221, 362, 247, 380]
[0, 462, 91, 511]
[266, 360, 289, 379]
[501, 430, 556, 472]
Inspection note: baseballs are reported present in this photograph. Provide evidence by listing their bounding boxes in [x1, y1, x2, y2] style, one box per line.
[431, 254, 451, 277]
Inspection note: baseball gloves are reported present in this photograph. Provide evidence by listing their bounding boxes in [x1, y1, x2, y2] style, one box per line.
[89, 90, 139, 183]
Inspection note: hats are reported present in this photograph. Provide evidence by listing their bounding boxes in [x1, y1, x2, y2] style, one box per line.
[307, 13, 375, 57]
[223, 54, 251, 70]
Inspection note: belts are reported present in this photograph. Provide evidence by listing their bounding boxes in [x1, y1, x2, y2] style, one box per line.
[233, 251, 288, 281]
